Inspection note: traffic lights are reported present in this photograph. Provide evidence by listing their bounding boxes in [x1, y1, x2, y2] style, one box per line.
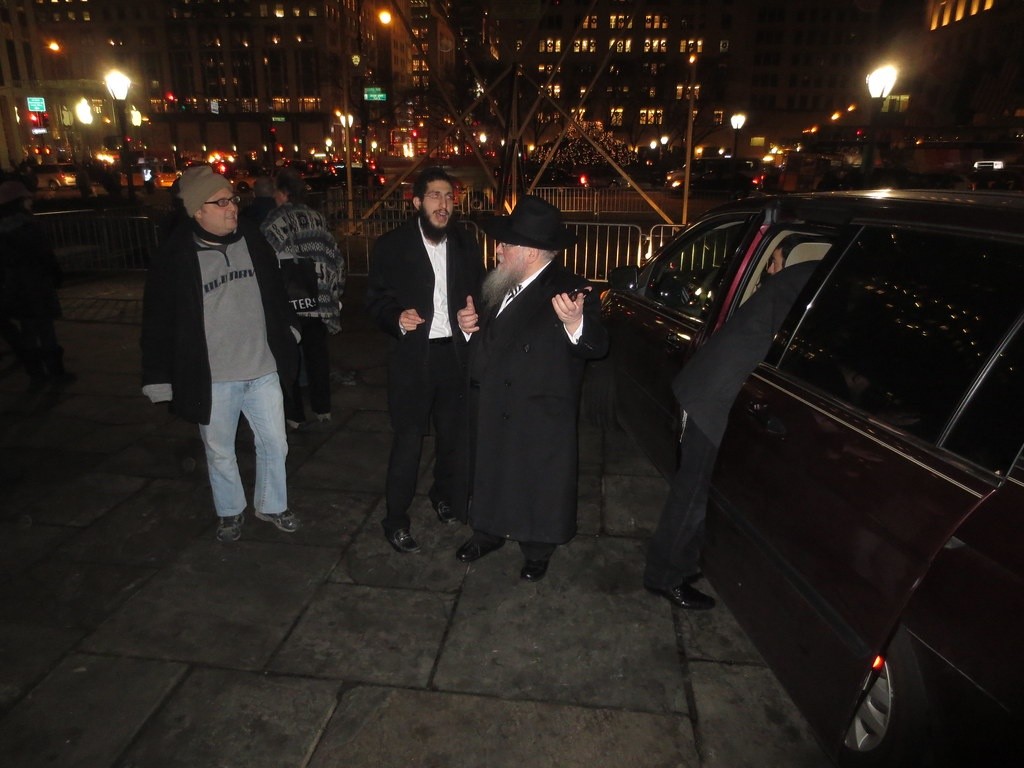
[31, 112, 50, 129]
[178, 103, 186, 112]
[123, 134, 130, 143]
[167, 92, 174, 102]
[268, 128, 276, 135]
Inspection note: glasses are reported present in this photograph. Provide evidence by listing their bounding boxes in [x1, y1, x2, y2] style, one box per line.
[204, 195, 241, 207]
[420, 192, 456, 200]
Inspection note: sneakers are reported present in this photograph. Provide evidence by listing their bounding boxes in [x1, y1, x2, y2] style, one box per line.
[216, 512, 245, 542]
[255, 507, 304, 532]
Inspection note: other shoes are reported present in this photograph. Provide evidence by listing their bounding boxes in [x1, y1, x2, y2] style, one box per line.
[315, 411, 332, 420]
[286, 416, 306, 428]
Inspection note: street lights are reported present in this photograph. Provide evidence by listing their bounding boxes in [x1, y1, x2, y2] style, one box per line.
[104, 67, 135, 203]
[864, 63, 901, 188]
[731, 114, 746, 157]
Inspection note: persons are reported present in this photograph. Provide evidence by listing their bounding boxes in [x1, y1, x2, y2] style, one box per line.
[261, 166, 346, 429]
[367, 166, 488, 553]
[768, 234, 813, 276]
[139, 166, 303, 542]
[643, 260, 821, 611]
[456, 195, 609, 582]
[240, 176, 277, 228]
[0, 181, 77, 383]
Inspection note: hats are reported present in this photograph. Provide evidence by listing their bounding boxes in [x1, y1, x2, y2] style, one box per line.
[481, 194, 579, 250]
[0, 181, 34, 204]
[180, 166, 234, 217]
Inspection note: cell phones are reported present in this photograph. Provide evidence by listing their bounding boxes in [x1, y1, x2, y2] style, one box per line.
[569, 288, 589, 302]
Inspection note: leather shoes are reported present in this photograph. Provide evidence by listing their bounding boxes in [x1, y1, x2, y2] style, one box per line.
[456, 533, 505, 563]
[521, 552, 548, 581]
[432, 498, 458, 524]
[384, 525, 421, 553]
[643, 577, 716, 610]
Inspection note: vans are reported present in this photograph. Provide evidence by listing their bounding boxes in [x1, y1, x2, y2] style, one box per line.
[582, 188, 1024, 768]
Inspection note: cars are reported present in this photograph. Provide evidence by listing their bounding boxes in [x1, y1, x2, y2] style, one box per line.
[37, 159, 384, 194]
[643, 154, 771, 199]
